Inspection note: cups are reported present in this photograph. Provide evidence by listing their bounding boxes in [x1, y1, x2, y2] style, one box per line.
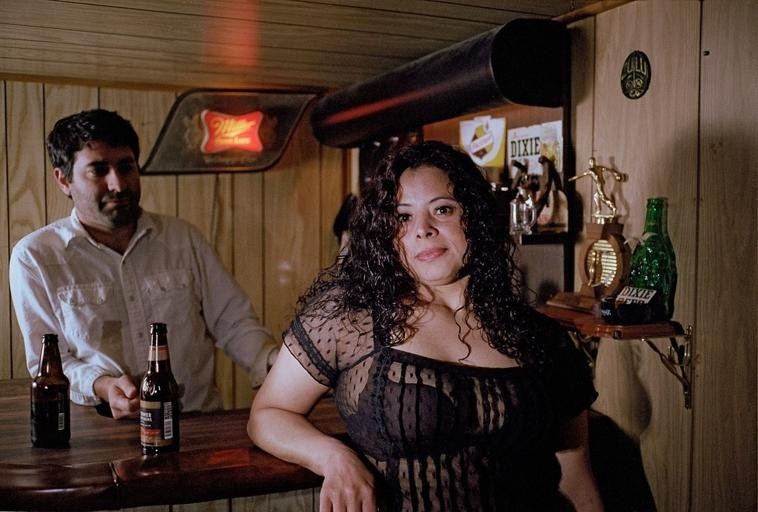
[507, 202, 538, 236]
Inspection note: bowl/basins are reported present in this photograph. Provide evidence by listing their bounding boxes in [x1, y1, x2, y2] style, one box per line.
[600, 297, 647, 326]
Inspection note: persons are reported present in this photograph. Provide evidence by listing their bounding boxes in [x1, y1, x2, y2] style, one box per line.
[246, 141, 605, 512]
[9, 109, 280, 421]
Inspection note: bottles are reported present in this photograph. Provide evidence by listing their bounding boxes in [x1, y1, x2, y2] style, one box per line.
[30, 334, 70, 448]
[627, 198, 671, 324]
[140, 323, 180, 456]
